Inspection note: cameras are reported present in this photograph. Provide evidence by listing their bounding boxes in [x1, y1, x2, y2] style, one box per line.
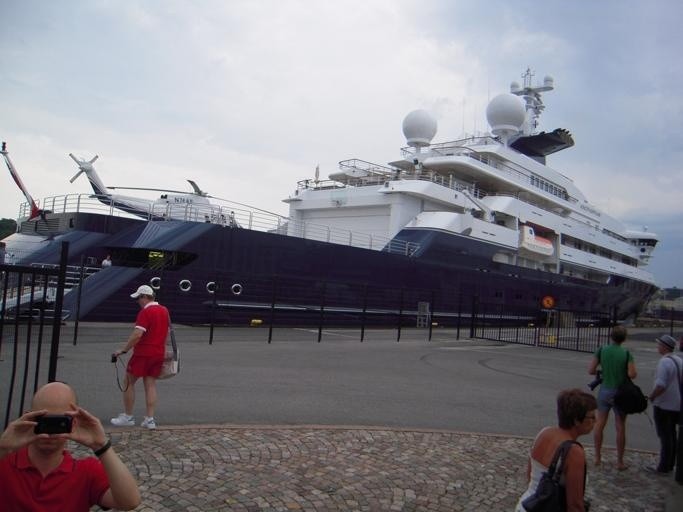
[111, 353, 116, 362]
[588, 369, 603, 391]
[34, 416, 73, 434]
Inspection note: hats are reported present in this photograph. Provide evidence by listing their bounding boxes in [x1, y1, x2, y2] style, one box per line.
[130, 285, 154, 298]
[656, 334, 677, 352]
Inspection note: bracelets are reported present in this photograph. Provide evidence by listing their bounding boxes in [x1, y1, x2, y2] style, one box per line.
[94, 439, 111, 457]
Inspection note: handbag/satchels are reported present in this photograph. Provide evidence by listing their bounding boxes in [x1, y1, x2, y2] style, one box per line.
[521, 441, 588, 512]
[156, 351, 180, 379]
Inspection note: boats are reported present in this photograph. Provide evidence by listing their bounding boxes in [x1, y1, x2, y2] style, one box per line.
[0, 62, 665, 329]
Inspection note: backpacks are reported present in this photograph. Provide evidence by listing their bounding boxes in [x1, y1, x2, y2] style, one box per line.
[614, 351, 648, 413]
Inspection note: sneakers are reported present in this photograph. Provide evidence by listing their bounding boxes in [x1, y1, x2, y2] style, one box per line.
[644, 464, 674, 474]
[615, 463, 632, 472]
[110, 416, 136, 427]
[593, 461, 602, 466]
[141, 419, 155, 430]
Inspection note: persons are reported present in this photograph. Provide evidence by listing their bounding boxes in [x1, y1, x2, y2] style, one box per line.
[110, 285, 171, 430]
[514, 388, 598, 512]
[0, 381, 142, 512]
[642, 334, 683, 475]
[588, 325, 637, 471]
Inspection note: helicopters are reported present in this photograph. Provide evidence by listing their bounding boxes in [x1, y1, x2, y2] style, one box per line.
[68, 151, 296, 229]
